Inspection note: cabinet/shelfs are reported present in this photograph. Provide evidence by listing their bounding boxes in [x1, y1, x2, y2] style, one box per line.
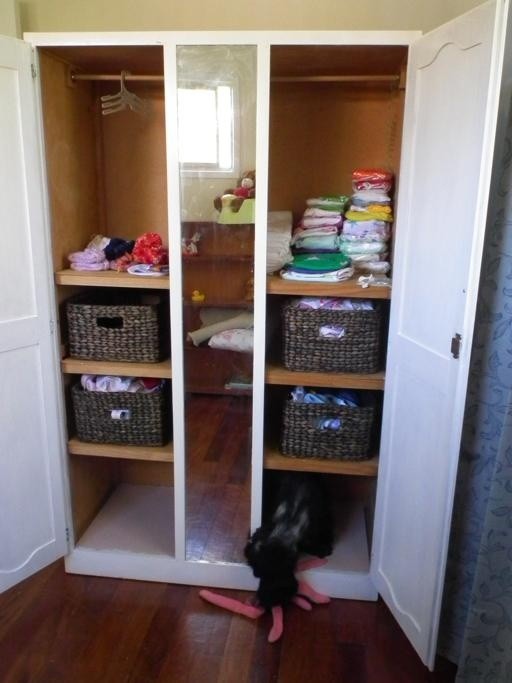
[0, 1, 512, 670]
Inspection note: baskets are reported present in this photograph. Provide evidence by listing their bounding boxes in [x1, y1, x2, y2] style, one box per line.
[279, 388, 377, 462]
[63, 290, 171, 362]
[67, 375, 170, 448]
[279, 297, 381, 373]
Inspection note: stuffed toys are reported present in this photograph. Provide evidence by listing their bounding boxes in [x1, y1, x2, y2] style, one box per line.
[213, 169, 256, 213]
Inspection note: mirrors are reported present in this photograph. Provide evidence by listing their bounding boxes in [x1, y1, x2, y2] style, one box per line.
[173, 40, 257, 571]
